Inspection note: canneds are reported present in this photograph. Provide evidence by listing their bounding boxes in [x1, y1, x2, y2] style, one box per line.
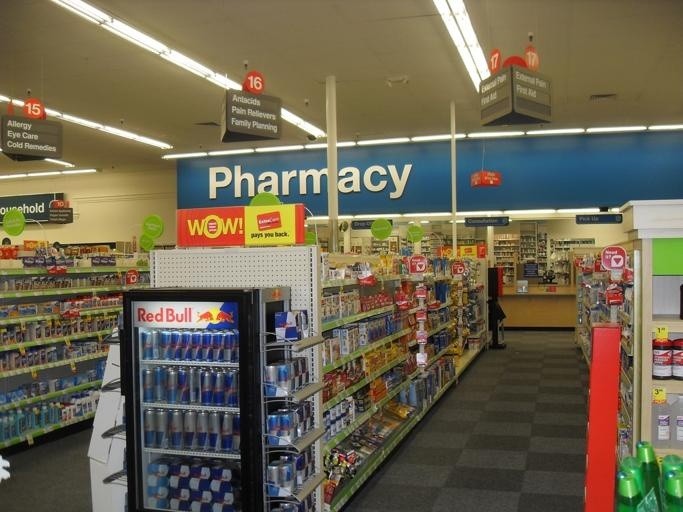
[267, 458, 296, 496]
[144, 409, 241, 452]
[673, 338, 683, 379]
[652, 339, 673, 380]
[264, 362, 291, 395]
[141, 329, 239, 363]
[143, 366, 239, 408]
[267, 411, 294, 445]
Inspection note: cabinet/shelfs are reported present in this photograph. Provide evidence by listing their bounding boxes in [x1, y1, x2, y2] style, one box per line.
[99, 230, 487, 512]
[0, 255, 149, 453]
[491, 209, 596, 327]
[575, 198, 683, 469]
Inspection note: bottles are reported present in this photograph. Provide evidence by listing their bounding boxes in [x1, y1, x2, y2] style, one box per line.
[617, 471, 641, 512]
[662, 471, 683, 512]
[637, 441, 660, 478]
[620, 456, 644, 497]
[661, 454, 683, 473]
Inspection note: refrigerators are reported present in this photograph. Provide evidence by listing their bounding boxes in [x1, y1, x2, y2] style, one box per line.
[123, 287, 292, 512]
